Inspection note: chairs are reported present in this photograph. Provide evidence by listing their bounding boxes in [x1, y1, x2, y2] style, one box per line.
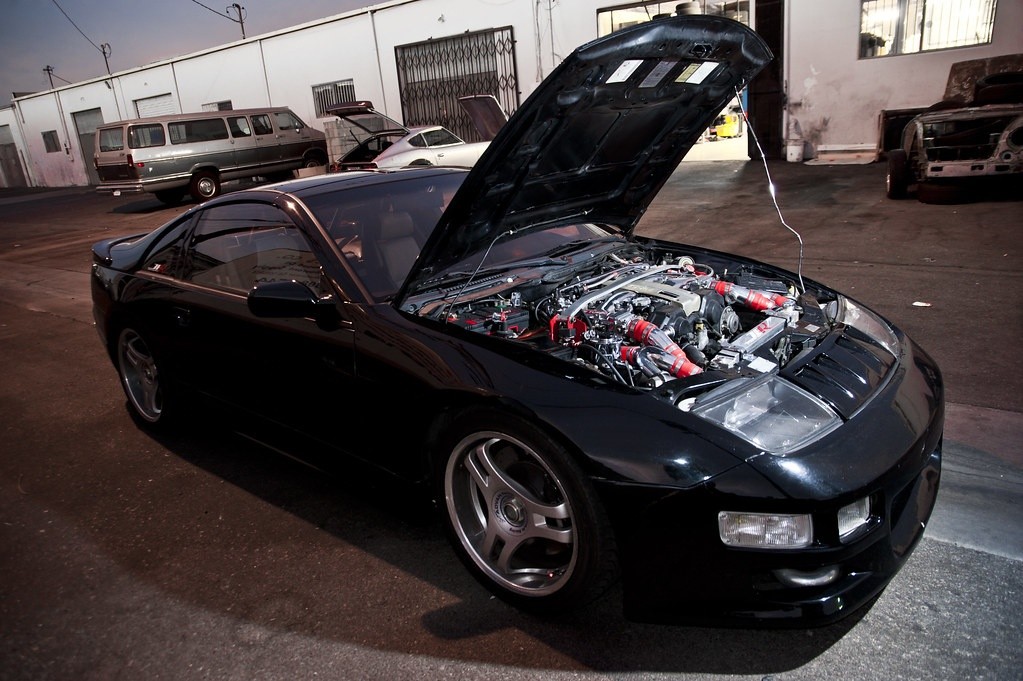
[149, 129, 165, 146]
[369, 211, 420, 292]
[382, 142, 393, 151]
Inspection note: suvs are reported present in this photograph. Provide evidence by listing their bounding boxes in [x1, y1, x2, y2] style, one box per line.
[886, 53, 1022, 202]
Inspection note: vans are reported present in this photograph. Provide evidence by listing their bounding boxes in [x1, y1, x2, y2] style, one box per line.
[93, 105, 326, 205]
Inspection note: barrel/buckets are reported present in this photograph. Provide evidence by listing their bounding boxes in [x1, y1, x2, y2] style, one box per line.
[786, 139, 804, 163]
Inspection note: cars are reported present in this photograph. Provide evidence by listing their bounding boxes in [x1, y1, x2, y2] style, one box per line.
[324, 94, 510, 173]
[90, 12, 946, 630]
